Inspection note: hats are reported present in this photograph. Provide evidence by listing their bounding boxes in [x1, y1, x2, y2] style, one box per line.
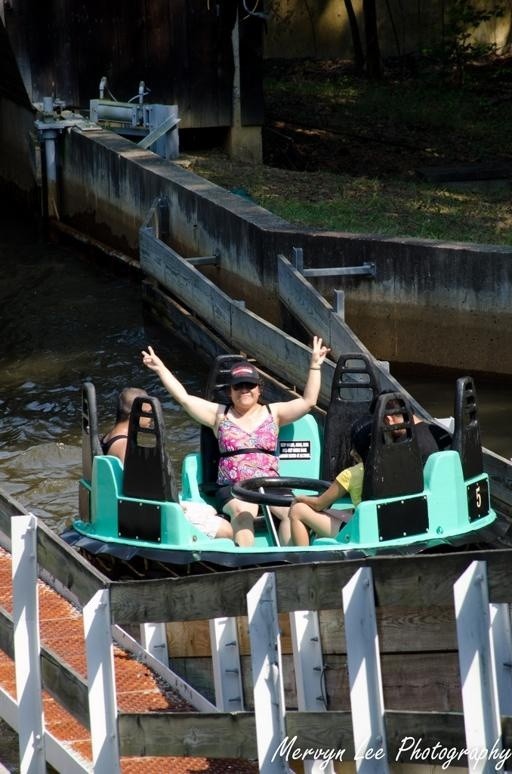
[228, 362, 261, 386]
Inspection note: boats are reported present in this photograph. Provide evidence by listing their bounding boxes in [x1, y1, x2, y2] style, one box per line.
[57, 350, 498, 568]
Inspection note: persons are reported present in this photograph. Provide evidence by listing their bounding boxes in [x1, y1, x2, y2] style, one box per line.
[370, 386, 453, 468]
[100, 386, 237, 541]
[139, 334, 334, 548]
[289, 413, 386, 547]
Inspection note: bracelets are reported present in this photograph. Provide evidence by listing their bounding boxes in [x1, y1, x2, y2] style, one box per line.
[308, 366, 322, 373]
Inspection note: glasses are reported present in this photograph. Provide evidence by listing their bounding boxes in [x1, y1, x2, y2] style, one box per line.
[232, 382, 258, 391]
